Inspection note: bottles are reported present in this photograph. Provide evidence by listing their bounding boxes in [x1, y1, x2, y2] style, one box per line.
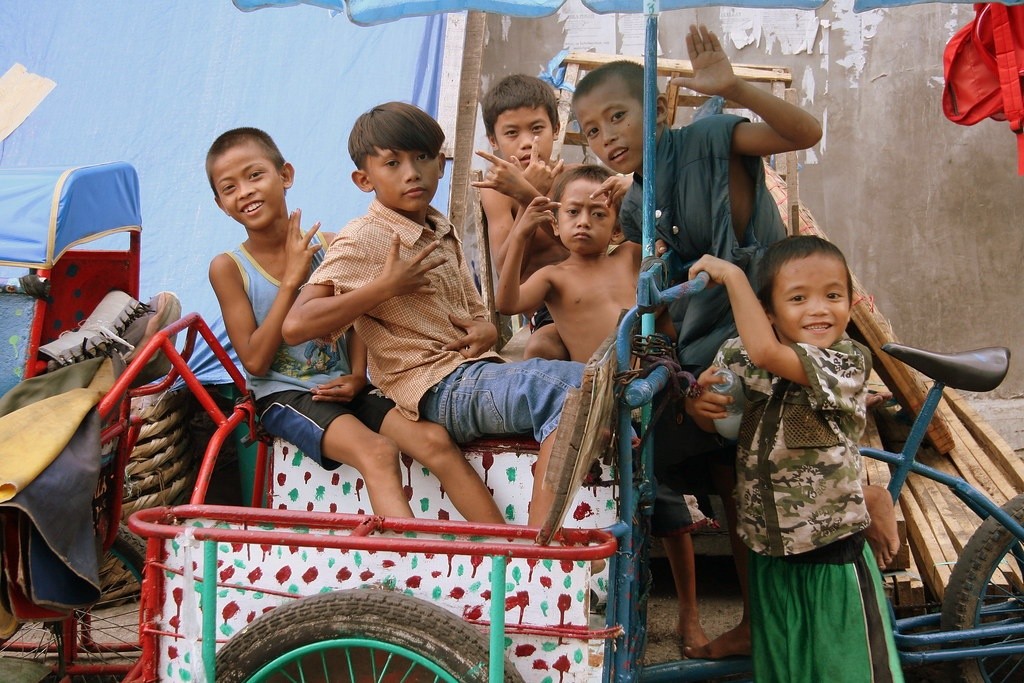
[707, 370, 746, 442]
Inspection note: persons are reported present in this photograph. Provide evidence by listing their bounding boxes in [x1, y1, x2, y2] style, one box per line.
[685, 235, 904, 683]
[495, 164, 711, 655]
[572, 25, 901, 568]
[282, 101, 635, 532]
[206, 126, 508, 525]
[475, 73, 569, 363]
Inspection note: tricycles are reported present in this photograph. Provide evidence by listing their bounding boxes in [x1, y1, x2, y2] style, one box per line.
[0, 1, 1024, 682]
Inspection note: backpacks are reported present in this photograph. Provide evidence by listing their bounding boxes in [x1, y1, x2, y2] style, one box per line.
[942, 3, 1024, 176]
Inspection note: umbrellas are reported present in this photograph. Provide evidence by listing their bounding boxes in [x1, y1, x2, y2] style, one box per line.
[236, 0, 1024, 512]
